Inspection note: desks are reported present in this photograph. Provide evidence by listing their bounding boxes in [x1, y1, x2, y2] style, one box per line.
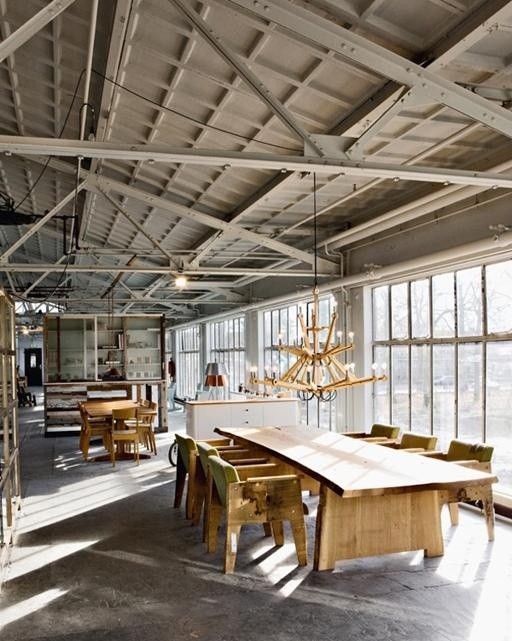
[213, 424, 499, 574]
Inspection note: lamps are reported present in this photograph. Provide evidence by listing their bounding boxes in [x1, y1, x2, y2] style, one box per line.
[249, 172, 389, 403]
[204, 358, 230, 401]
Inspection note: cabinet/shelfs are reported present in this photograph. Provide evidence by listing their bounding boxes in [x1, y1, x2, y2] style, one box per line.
[43, 313, 162, 383]
[184, 398, 300, 446]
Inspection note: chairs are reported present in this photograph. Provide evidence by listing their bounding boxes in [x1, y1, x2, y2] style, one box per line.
[173, 433, 309, 575]
[77, 398, 157, 468]
[338, 424, 496, 542]
